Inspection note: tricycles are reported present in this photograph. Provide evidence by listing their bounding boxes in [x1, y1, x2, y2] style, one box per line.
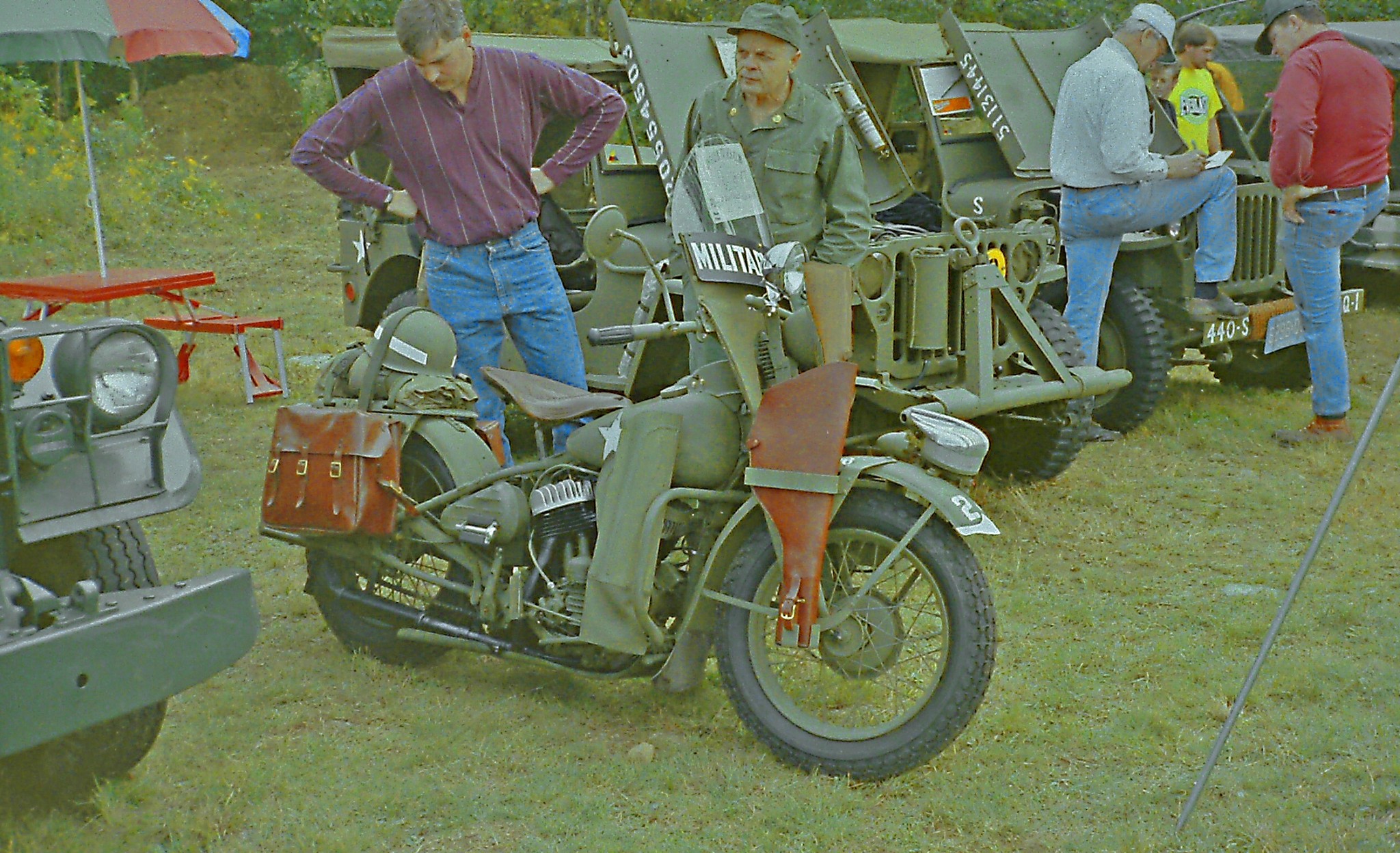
[311, 3, 1400, 482]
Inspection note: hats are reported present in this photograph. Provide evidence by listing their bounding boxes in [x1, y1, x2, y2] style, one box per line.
[1255, 0, 1325, 57]
[1130, 3, 1177, 64]
[727, 2, 801, 50]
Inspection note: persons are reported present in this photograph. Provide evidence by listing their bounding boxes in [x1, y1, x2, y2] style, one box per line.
[291, 0, 628, 469]
[1168, 23, 1223, 158]
[1255, 0, 1392, 446]
[1049, 2, 1251, 442]
[1147, 59, 1181, 129]
[664, 2, 871, 375]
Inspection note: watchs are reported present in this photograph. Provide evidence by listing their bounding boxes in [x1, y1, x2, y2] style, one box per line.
[384, 189, 393, 209]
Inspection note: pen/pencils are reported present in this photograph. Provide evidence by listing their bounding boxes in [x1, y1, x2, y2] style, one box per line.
[1191, 140, 1198, 151]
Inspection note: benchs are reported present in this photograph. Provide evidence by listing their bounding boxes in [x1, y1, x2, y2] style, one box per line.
[144, 315, 289, 404]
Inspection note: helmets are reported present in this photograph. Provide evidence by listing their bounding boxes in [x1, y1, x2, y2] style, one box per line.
[363, 306, 458, 379]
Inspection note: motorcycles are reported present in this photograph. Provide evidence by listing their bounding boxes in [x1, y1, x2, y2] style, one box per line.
[255, 132, 1004, 783]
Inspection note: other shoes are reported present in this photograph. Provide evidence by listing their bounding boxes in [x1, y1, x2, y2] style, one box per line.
[1271, 415, 1355, 448]
[1187, 290, 1251, 322]
[1081, 420, 1123, 442]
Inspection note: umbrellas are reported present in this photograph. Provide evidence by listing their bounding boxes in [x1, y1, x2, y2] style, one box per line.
[0, 0, 250, 317]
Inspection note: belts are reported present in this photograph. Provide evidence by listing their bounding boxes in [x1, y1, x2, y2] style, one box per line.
[1298, 176, 1387, 203]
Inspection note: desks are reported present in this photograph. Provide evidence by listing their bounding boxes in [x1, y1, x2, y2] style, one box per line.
[0, 268, 218, 318]
